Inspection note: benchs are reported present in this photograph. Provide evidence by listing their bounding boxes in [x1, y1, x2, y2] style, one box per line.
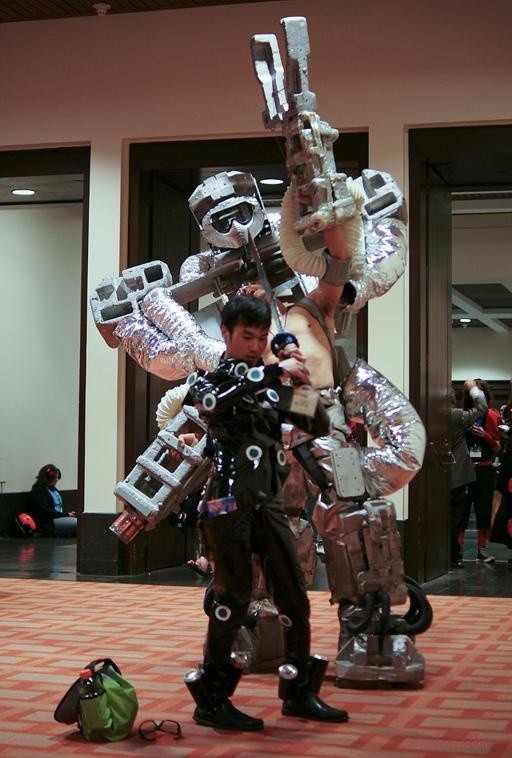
[0, 491, 76, 538]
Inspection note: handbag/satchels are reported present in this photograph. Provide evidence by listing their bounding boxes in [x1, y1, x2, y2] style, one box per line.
[54, 657, 137, 743]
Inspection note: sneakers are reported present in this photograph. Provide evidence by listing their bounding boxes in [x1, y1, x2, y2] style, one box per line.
[477, 547, 495, 562]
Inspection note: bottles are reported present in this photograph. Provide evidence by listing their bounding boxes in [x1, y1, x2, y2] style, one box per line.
[79, 664, 110, 730]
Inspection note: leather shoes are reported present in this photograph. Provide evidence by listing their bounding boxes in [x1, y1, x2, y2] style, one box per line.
[193, 701, 263, 731]
[281, 696, 349, 722]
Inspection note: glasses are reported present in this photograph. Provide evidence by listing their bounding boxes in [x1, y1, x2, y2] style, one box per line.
[139, 719, 181, 741]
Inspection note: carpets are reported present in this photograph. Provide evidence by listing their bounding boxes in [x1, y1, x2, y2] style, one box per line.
[1, 577, 512, 758]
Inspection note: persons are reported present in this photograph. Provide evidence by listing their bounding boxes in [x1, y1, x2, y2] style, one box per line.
[169, 227, 430, 513]
[451, 377, 512, 569]
[29, 464, 77, 540]
[182, 292, 350, 731]
